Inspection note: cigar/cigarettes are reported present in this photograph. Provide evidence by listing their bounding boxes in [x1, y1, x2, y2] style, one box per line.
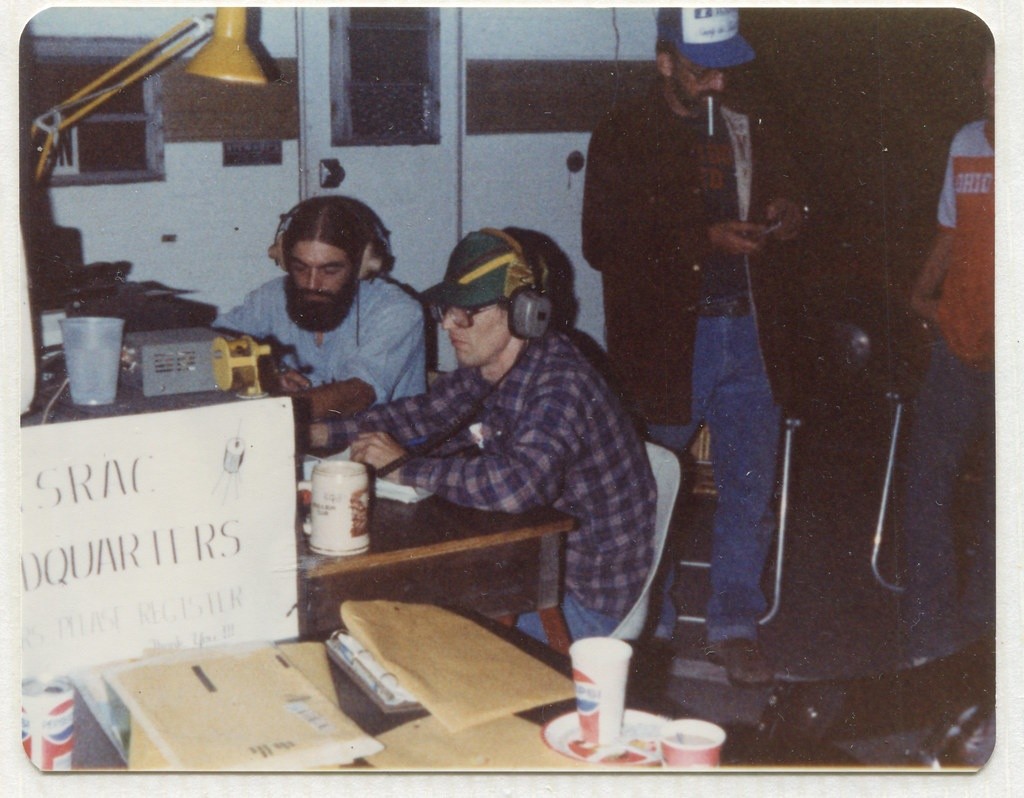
[706, 96, 715, 136]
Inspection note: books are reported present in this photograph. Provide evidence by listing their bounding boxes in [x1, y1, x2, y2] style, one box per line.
[323, 627, 427, 714]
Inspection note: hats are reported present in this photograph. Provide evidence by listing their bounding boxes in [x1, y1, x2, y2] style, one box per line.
[421, 228, 548, 307]
[655, 7, 757, 68]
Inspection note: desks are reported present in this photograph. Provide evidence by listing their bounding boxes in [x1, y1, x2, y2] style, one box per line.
[297, 492, 575, 640]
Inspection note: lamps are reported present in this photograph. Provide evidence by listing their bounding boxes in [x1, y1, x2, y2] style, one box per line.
[29, 7, 271, 182]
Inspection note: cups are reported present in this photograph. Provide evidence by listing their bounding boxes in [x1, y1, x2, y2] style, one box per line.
[564, 635, 632, 746]
[657, 717, 727, 768]
[55, 314, 127, 408]
[298, 457, 373, 556]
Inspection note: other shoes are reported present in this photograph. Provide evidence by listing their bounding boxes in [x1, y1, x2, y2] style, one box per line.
[647, 629, 772, 688]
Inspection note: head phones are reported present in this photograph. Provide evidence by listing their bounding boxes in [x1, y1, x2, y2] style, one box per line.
[268, 195, 383, 283]
[502, 232, 552, 336]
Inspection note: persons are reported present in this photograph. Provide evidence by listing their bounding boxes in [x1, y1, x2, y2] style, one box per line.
[905, 35, 995, 644]
[296, 228, 662, 656]
[208, 196, 428, 422]
[577, 8, 815, 692]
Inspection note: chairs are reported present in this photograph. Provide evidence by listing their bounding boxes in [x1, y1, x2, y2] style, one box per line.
[675, 407, 803, 629]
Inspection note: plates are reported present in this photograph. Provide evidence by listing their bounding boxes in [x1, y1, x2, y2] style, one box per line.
[538, 708, 670, 770]
